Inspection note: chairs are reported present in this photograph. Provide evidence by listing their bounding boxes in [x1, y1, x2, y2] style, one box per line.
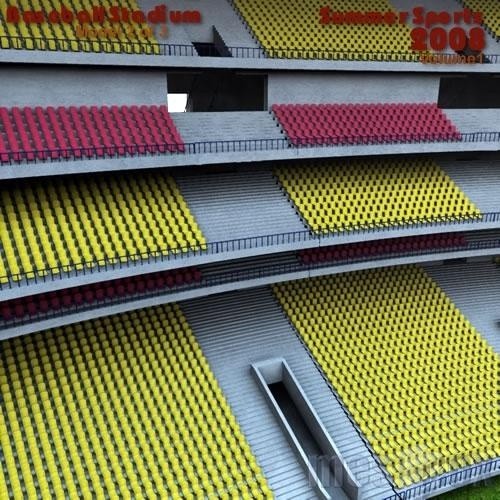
[0, 105, 185, 163]
[270, 102, 463, 148]
[228, 0, 438, 63]
[0, 265, 203, 322]
[0, 0, 162, 56]
[458, 0, 500, 43]
[1, 301, 274, 500]
[0, 167, 207, 285]
[270, 264, 500, 489]
[267, 154, 483, 235]
[295, 232, 467, 265]
